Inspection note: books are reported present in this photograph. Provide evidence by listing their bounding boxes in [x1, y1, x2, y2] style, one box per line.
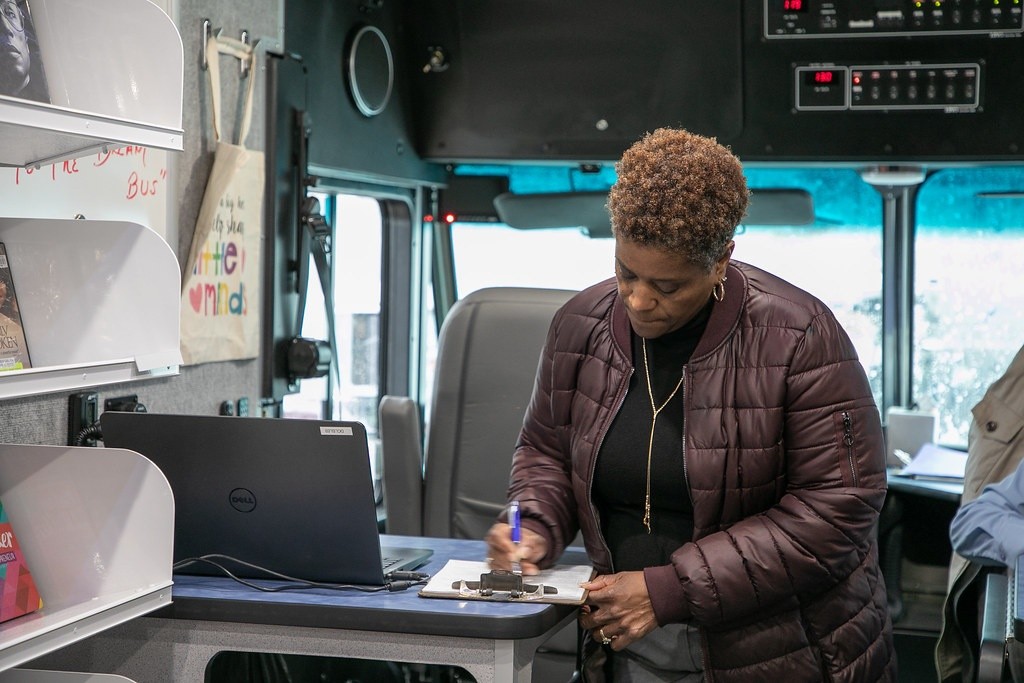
[0, 501, 43, 624]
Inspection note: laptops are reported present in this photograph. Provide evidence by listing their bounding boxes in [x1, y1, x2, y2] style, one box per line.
[101, 411, 434, 584]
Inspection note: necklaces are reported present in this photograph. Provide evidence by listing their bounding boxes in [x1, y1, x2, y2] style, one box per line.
[640, 337, 685, 537]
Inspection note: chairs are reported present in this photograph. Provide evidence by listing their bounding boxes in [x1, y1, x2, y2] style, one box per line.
[378, 286, 585, 550]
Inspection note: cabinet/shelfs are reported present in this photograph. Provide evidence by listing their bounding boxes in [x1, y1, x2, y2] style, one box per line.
[0, 2, 185, 683]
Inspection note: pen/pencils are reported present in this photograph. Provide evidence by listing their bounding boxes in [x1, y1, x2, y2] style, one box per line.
[507, 501, 523, 577]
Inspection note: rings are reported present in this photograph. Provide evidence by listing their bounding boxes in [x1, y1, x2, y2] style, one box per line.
[597, 629, 612, 646]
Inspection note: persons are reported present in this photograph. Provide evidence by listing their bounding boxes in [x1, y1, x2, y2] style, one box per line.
[0, 0, 50, 103]
[946, 458, 1024, 571]
[488, 126, 904, 683]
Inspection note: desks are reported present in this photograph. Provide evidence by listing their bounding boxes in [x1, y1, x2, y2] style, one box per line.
[17, 532, 596, 683]
